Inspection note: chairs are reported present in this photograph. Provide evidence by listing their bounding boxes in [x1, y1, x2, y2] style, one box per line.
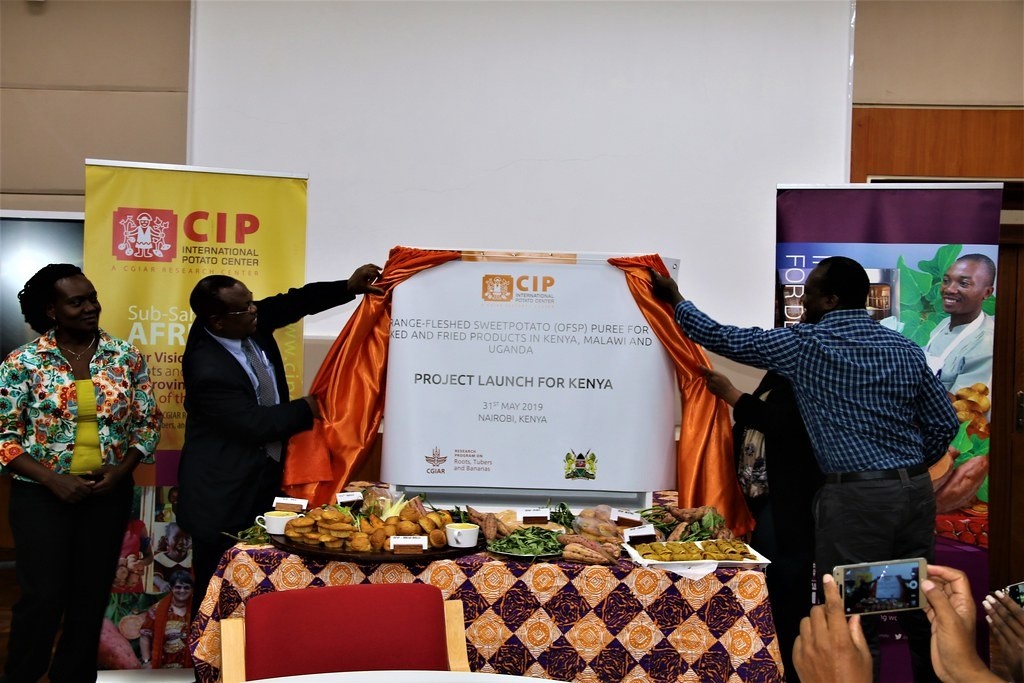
[219, 583, 469, 683]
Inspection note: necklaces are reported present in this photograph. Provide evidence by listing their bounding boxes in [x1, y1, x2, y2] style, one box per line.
[56, 336, 95, 361]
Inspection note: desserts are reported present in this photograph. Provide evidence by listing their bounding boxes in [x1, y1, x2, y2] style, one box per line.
[284, 508, 452, 553]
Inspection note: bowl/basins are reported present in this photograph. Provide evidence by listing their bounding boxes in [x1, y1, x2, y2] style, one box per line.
[255, 511, 305, 535]
[445, 523, 480, 548]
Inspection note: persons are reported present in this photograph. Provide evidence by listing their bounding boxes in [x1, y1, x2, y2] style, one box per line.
[982, 590, 1024, 668]
[1, 263, 161, 683]
[923, 253, 994, 420]
[177, 263, 384, 610]
[693, 303, 819, 683]
[792, 565, 1005, 683]
[648, 255, 960, 683]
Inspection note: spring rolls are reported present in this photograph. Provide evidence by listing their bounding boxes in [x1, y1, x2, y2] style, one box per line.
[635, 540, 757, 560]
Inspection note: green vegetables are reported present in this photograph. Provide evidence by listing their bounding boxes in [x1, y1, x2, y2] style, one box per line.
[489, 503, 576, 556]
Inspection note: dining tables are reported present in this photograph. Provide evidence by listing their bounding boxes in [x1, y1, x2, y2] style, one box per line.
[187, 502, 786, 681]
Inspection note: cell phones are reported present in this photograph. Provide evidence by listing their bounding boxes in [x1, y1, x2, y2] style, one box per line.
[1006, 581, 1024, 608]
[832, 557, 928, 616]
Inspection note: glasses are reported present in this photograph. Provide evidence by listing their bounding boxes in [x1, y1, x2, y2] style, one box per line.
[217, 292, 253, 316]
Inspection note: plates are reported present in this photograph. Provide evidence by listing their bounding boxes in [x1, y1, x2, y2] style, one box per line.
[634, 539, 772, 568]
[486, 546, 561, 556]
[269, 533, 486, 562]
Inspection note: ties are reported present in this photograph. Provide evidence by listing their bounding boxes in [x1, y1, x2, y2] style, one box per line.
[241, 335, 281, 463]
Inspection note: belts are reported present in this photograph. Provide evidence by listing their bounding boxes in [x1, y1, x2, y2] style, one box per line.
[824, 462, 929, 485]
